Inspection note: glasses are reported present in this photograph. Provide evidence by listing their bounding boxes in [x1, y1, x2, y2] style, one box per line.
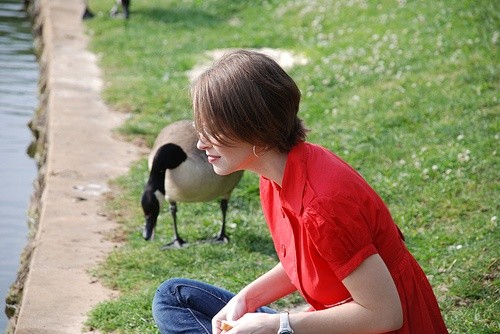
[192, 122, 215, 137]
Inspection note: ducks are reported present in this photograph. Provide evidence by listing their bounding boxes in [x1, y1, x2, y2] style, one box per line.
[141, 119, 245, 250]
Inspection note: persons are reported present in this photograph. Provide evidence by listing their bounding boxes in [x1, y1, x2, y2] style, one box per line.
[152, 50, 449, 334]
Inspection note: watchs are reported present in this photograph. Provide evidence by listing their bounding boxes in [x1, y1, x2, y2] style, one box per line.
[277, 311, 294, 334]
